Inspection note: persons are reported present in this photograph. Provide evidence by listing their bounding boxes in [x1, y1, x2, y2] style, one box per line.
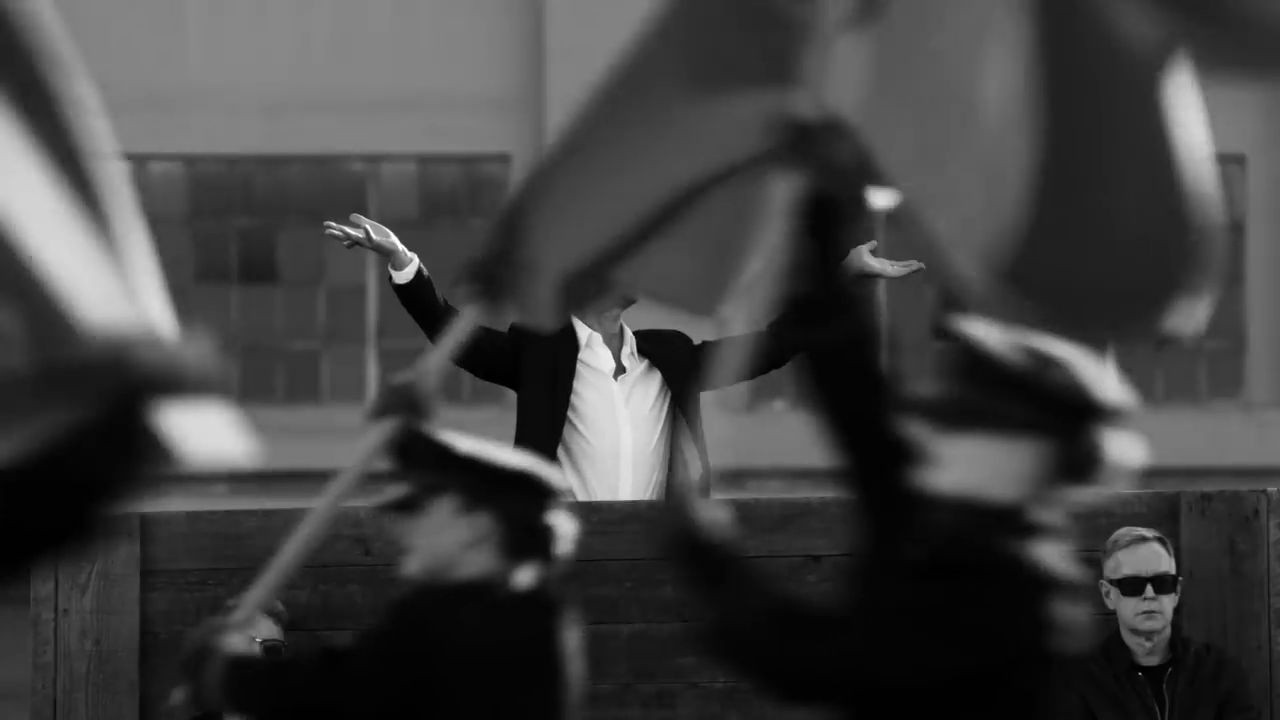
[320, 211, 925, 502]
[1059, 523, 1255, 720]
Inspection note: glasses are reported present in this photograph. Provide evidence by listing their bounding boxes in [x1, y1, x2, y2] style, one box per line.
[1108, 574, 1179, 597]
